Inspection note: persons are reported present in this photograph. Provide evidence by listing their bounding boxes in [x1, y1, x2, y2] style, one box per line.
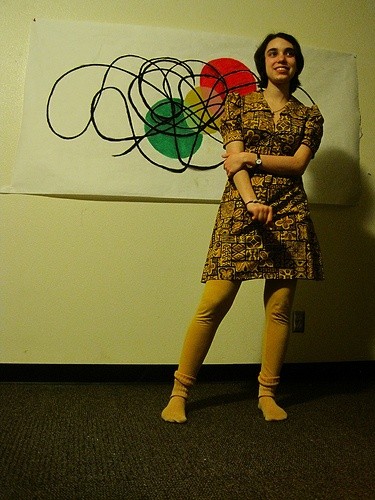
[161, 32, 325, 424]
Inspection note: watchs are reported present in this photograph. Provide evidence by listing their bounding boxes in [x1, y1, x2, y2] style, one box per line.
[255, 154, 262, 168]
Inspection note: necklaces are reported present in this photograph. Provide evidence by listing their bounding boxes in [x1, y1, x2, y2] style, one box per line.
[262, 96, 289, 115]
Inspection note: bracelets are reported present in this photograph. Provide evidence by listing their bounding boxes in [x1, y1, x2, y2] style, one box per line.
[245, 200, 262, 207]
